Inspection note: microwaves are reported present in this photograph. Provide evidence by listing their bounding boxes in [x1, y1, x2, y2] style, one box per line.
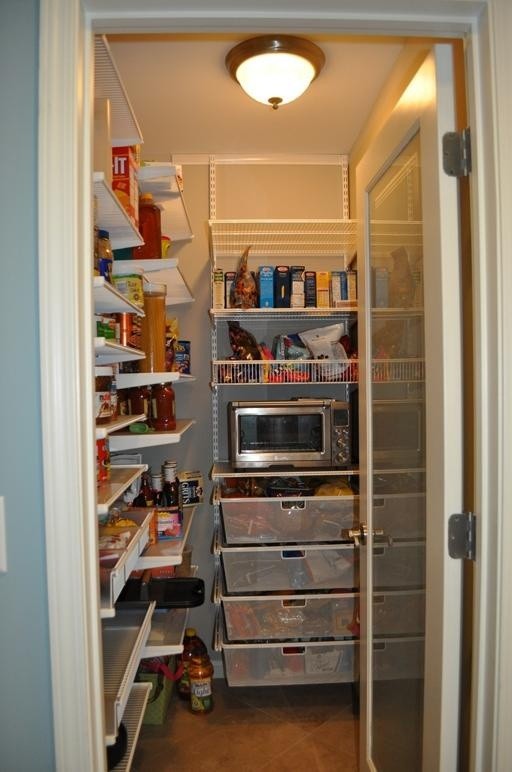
[226, 394, 352, 473]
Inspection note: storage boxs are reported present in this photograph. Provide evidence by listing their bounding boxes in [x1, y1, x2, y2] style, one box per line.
[371, 492, 426, 681]
[216, 488, 359, 689]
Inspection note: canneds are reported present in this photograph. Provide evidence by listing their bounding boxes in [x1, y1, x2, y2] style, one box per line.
[117, 382, 175, 430]
[91, 366, 120, 426]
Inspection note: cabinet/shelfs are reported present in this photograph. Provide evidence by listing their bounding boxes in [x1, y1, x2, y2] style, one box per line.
[369, 220, 426, 475]
[210, 219, 359, 478]
[94, 34, 198, 772]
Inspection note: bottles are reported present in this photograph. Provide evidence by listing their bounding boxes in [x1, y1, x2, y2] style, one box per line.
[90, 186, 215, 718]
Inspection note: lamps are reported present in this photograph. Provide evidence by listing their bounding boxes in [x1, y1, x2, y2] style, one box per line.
[224, 34, 326, 110]
